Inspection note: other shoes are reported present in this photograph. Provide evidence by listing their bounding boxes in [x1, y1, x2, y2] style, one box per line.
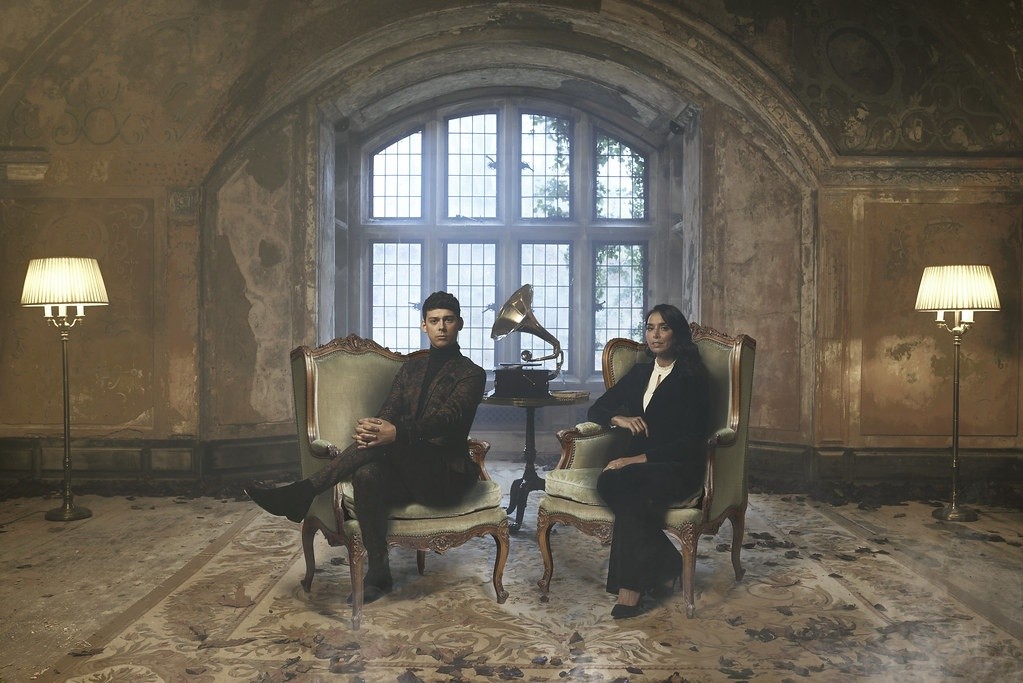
[348, 570, 393, 606]
[244, 481, 313, 523]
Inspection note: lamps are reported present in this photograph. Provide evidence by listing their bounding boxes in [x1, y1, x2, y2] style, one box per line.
[915, 265, 1003, 522]
[22, 256, 111, 523]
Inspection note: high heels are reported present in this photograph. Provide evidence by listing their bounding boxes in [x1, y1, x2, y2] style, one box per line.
[611, 587, 648, 617]
[650, 556, 684, 600]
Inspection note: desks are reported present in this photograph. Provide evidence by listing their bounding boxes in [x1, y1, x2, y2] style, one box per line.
[479, 391, 590, 535]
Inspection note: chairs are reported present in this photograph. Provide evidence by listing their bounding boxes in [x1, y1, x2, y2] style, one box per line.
[291, 333, 509, 631]
[535, 322, 757, 618]
